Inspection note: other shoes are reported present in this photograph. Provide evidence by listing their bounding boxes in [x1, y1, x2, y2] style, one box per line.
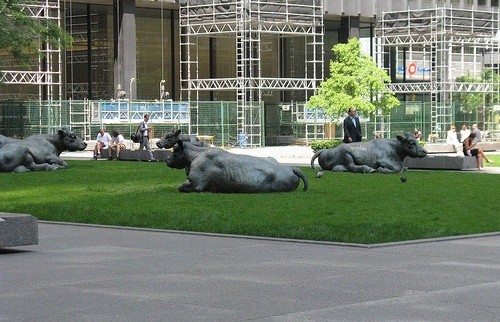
[148, 158, 159, 162]
[113, 157, 120, 161]
[90, 156, 97, 160]
[478, 168, 485, 171]
[486, 159, 494, 163]
[96, 153, 103, 158]
[105, 157, 113, 160]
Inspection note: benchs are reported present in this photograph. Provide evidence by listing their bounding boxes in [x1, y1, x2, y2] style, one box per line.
[101, 135, 213, 162]
[402, 142, 496, 171]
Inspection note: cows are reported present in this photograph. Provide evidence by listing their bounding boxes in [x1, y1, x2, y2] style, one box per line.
[0, 125, 87, 173]
[164, 139, 308, 194]
[311, 134, 427, 174]
[156, 129, 211, 149]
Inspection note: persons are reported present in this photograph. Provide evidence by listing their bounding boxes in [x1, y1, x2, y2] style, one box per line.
[463, 132, 494, 170]
[413, 128, 423, 141]
[137, 114, 156, 162]
[343, 107, 364, 143]
[91, 128, 112, 161]
[108, 130, 126, 162]
[446, 124, 482, 155]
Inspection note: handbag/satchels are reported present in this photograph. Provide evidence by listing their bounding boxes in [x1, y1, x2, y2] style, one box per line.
[131, 132, 143, 143]
